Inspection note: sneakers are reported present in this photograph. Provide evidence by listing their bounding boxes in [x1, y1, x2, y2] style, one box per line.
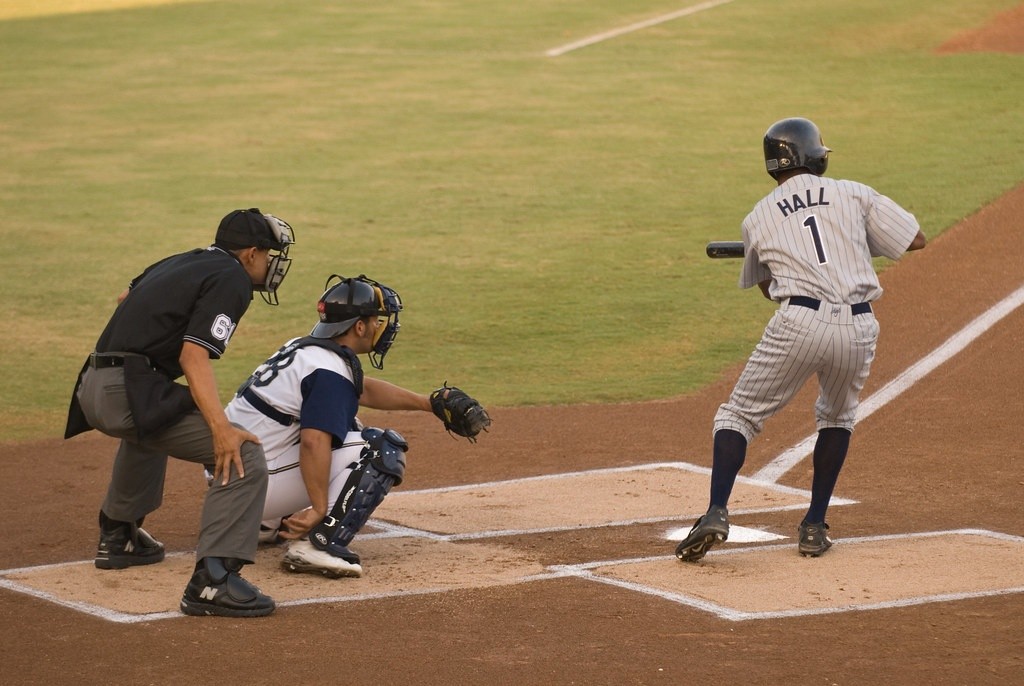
[94, 522, 165, 569]
[280, 540, 362, 579]
[674, 505, 729, 562]
[180, 558, 276, 617]
[258, 525, 289, 544]
[798, 520, 832, 557]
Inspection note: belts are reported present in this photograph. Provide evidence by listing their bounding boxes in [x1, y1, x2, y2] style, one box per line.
[89, 356, 159, 369]
[789, 296, 872, 315]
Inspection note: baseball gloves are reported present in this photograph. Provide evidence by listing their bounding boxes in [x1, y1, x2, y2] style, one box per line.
[429, 380, 492, 438]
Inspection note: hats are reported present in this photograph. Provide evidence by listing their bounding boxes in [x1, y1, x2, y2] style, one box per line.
[215, 210, 283, 251]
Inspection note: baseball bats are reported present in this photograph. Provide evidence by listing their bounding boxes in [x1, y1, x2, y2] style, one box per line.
[705, 240, 745, 259]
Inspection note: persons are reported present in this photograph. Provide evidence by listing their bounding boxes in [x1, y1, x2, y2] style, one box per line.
[64, 208, 296, 618]
[205, 274, 493, 576]
[674, 118, 926, 563]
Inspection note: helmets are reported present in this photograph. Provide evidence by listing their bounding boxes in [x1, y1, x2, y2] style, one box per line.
[310, 280, 381, 338]
[763, 117, 833, 180]
